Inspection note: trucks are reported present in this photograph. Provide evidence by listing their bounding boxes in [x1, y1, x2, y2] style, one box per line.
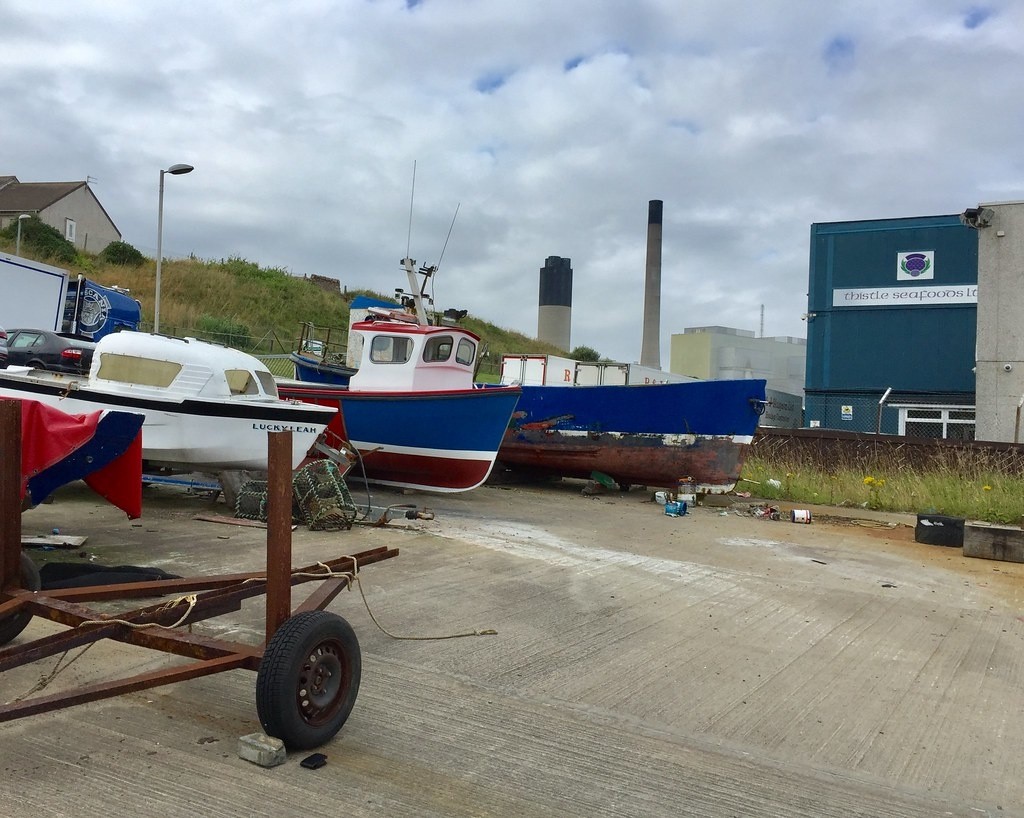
[499, 352, 582, 387]
[573, 359, 706, 386]
[0, 251, 143, 348]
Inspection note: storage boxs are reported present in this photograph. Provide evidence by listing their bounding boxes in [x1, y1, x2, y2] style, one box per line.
[915, 514, 966, 547]
[963, 522, 1024, 563]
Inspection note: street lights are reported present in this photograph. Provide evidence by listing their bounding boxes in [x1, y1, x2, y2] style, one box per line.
[154, 163, 195, 335]
[16, 214, 31, 256]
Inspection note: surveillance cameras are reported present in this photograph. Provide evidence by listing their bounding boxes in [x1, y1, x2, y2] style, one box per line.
[971, 366, 976, 374]
[1004, 364, 1011, 372]
[801, 313, 806, 320]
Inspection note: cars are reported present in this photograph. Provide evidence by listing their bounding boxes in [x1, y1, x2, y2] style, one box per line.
[0, 325, 9, 369]
[5, 328, 97, 375]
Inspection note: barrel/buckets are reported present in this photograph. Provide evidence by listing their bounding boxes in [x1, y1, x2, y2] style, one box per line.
[655, 492, 667, 504]
[666, 501, 687, 516]
[677, 478, 697, 508]
[790, 508, 812, 524]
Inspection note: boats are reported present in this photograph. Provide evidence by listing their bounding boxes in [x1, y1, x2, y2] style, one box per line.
[288, 320, 774, 496]
[270, 161, 524, 493]
[0, 328, 338, 508]
